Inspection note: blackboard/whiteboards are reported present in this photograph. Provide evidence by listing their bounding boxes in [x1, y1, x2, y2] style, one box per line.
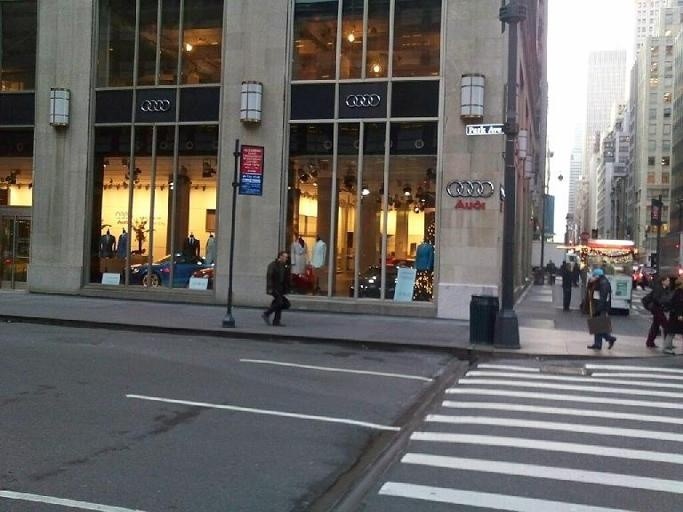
[393, 269, 417, 302]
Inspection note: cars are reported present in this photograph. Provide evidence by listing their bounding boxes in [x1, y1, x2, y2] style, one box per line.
[125, 251, 213, 290]
[631, 260, 655, 289]
[188, 252, 316, 296]
[348, 256, 432, 302]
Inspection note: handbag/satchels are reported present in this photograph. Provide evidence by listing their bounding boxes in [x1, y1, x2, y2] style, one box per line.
[587, 318, 611, 334]
[643, 292, 653, 310]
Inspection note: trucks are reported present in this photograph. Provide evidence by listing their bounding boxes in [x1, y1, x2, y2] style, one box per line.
[648, 232, 682, 282]
[531, 240, 580, 274]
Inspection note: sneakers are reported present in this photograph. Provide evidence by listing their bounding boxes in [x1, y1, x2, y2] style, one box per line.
[586, 344, 601, 349]
[273, 320, 285, 326]
[646, 341, 676, 354]
[261, 312, 271, 326]
[608, 337, 615, 349]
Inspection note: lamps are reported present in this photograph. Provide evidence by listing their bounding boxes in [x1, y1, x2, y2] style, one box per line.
[458, 72, 486, 121]
[294, 158, 435, 214]
[4, 156, 217, 186]
[47, 87, 71, 131]
[239, 80, 262, 129]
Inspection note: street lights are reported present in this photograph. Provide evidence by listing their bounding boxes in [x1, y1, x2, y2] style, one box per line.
[538, 171, 564, 272]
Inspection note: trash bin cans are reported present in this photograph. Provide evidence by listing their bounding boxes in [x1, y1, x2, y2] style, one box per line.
[533, 270, 544, 285]
[470, 296, 499, 346]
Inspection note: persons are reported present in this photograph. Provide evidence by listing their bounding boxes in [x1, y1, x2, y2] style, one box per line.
[391, 251, 396, 260]
[411, 51, 437, 77]
[543, 261, 683, 355]
[100, 229, 115, 258]
[296, 51, 318, 80]
[205, 234, 217, 263]
[261, 250, 291, 327]
[387, 254, 391, 259]
[183, 234, 200, 258]
[116, 230, 128, 259]
[309, 235, 327, 268]
[416, 242, 434, 272]
[290, 236, 307, 274]
